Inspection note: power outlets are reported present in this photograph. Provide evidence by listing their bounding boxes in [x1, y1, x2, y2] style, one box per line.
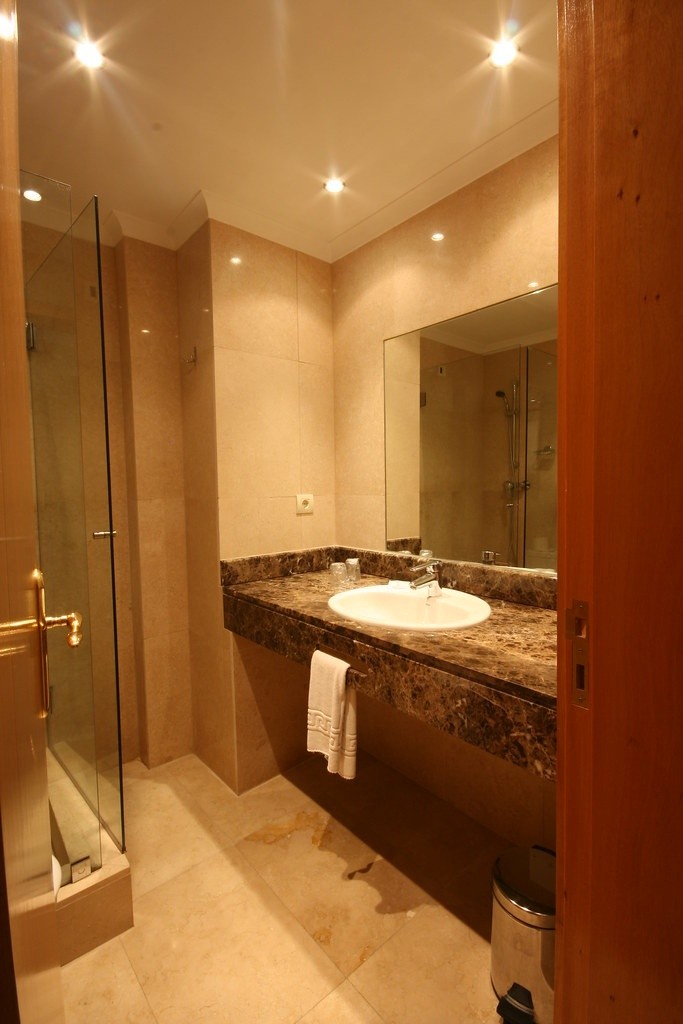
[297, 492, 315, 515]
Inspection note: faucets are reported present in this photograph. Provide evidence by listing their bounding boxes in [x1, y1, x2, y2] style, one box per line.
[480, 550, 502, 565]
[409, 559, 443, 598]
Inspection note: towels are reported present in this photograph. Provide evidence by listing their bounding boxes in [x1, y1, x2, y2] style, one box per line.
[305, 648, 358, 781]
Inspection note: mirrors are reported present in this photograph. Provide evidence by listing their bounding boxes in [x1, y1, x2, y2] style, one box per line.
[382, 280, 560, 575]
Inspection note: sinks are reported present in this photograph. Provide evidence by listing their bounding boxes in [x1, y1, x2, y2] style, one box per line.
[327, 583, 492, 632]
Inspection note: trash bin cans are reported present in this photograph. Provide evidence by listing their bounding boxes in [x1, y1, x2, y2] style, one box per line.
[484, 832, 558, 1023]
